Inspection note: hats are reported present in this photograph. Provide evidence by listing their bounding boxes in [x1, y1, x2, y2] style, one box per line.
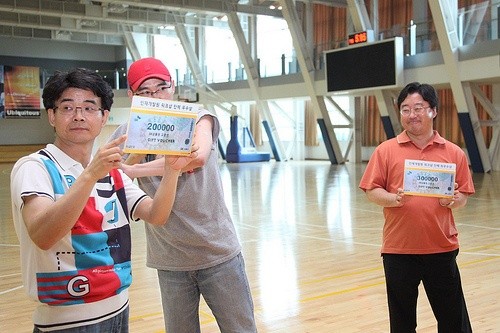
[127, 57, 171, 92]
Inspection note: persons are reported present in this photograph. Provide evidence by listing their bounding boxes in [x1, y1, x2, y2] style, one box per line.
[106, 57, 258, 333]
[9, 66, 201, 333]
[356, 81, 476, 333]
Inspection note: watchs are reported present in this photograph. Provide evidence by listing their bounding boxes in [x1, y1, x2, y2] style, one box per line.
[440, 200, 455, 207]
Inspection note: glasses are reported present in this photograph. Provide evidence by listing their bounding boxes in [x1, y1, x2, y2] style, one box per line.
[399, 105, 431, 116]
[132, 84, 172, 98]
[52, 104, 104, 116]
